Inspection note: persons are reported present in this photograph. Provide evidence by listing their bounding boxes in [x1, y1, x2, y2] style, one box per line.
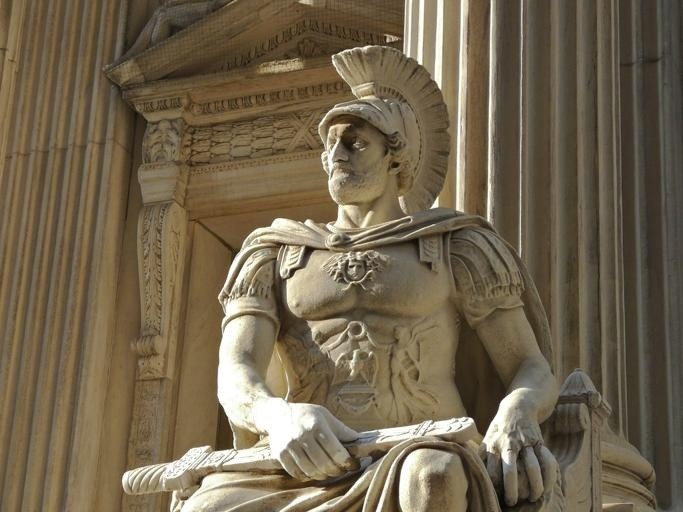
[178, 46, 558, 511]
